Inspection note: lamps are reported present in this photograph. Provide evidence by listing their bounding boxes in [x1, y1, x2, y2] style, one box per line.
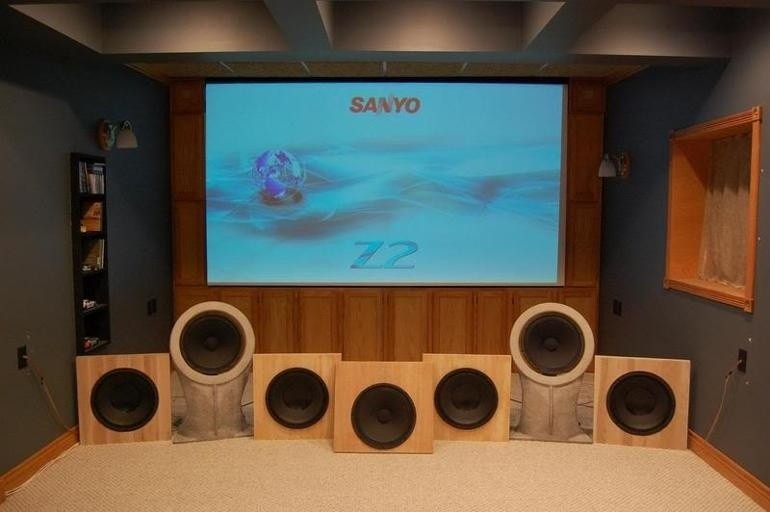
[99, 117, 139, 152]
[597, 150, 629, 179]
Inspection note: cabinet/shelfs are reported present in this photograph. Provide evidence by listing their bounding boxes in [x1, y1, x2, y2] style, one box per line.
[71, 152, 111, 355]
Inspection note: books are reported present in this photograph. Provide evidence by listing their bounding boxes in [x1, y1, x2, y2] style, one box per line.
[78, 160, 108, 196]
[72, 200, 103, 232]
[83, 238, 106, 272]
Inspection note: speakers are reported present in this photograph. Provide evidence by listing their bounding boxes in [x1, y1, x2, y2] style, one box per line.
[253, 353, 343, 442]
[76, 353, 171, 444]
[510, 301, 595, 445]
[595, 354, 691, 450]
[422, 351, 512, 444]
[169, 300, 255, 444]
[332, 360, 434, 455]
